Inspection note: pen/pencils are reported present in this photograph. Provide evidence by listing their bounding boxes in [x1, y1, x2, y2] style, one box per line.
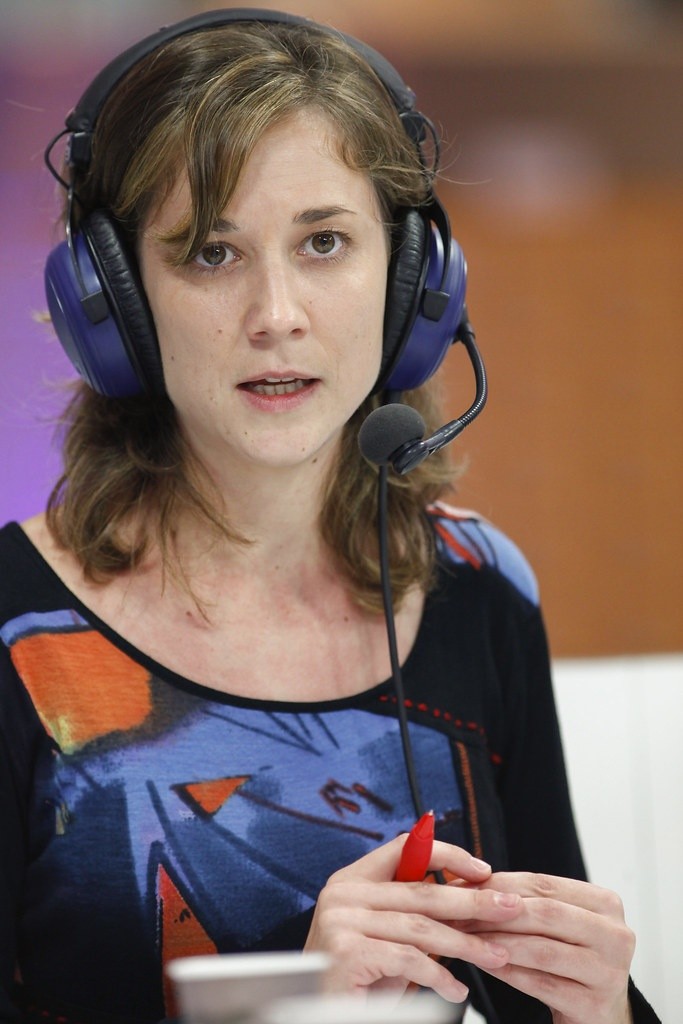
[395, 808, 438, 884]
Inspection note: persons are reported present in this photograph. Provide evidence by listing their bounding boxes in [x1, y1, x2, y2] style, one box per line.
[0, 8, 659, 1024]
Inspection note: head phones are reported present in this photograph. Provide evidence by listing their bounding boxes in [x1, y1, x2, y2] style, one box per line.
[42, 3, 468, 412]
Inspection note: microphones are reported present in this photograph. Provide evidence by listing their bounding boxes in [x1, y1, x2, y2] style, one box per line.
[359, 320, 489, 476]
